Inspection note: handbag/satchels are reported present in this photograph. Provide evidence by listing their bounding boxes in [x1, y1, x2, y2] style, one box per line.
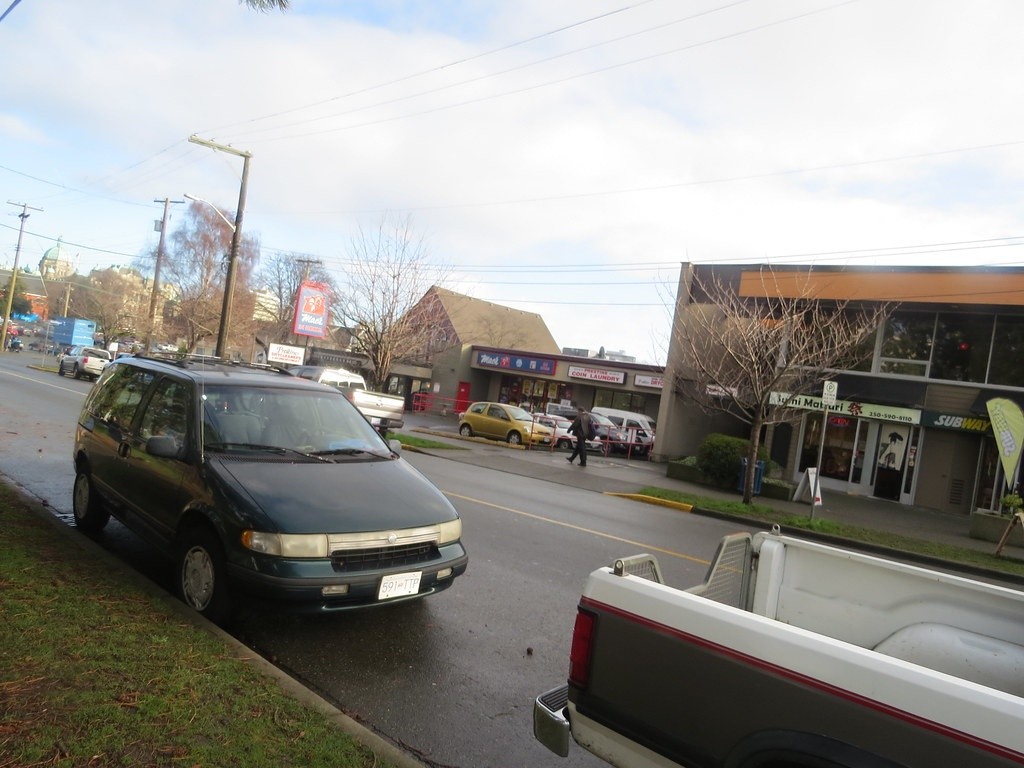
[587, 423, 596, 440]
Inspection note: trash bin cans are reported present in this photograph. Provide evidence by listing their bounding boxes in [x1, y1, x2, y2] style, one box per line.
[738, 456, 766, 495]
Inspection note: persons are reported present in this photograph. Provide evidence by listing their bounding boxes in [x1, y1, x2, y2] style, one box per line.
[57, 347, 64, 365]
[565, 406, 593, 467]
[5, 336, 11, 352]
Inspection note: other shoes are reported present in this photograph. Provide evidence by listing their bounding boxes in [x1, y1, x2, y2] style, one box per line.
[566, 457, 572, 462]
[577, 464, 586, 466]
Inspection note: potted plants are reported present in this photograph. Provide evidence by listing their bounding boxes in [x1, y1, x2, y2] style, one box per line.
[766, 461, 783, 479]
[971, 495, 1024, 548]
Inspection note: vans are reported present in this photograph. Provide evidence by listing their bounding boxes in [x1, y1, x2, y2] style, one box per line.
[590, 406, 656, 456]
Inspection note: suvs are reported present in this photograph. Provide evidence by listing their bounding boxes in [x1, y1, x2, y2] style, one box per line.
[69, 351, 471, 624]
[550, 408, 644, 455]
[457, 401, 553, 447]
[101, 352, 154, 382]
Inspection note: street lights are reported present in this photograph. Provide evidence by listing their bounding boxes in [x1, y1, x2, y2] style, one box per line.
[183, 192, 246, 360]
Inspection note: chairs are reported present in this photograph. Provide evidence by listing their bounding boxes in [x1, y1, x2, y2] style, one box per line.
[215, 410, 261, 446]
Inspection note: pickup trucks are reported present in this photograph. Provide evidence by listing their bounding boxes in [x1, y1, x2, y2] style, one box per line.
[281, 365, 406, 440]
[531, 521, 1024, 768]
[57, 345, 113, 381]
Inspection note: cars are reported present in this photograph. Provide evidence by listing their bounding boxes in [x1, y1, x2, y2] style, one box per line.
[0, 310, 55, 354]
[94, 332, 180, 358]
[515, 412, 603, 450]
